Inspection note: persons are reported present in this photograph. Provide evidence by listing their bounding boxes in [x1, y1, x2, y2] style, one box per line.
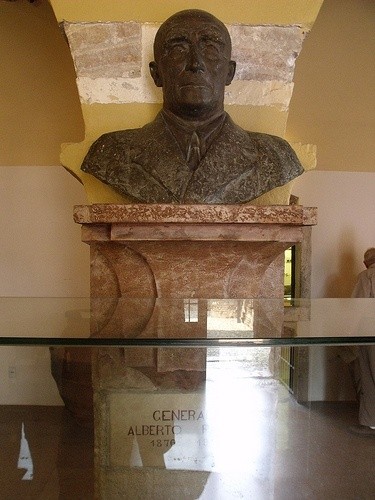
[345, 247, 374, 439]
[80, 8, 305, 204]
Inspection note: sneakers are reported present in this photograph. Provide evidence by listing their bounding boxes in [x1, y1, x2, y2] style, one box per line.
[346, 426, 375, 438]
